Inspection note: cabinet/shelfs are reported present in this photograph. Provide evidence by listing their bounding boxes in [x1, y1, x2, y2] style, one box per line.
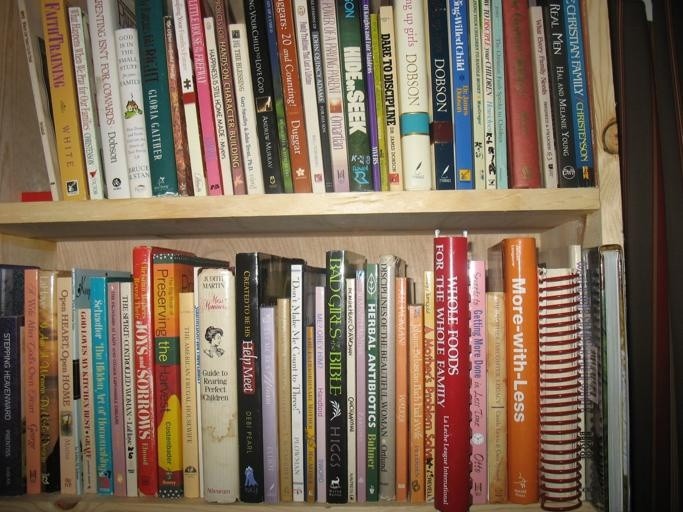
[0, 1, 659, 512]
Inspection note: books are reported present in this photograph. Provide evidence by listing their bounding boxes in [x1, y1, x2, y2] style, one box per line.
[18, 1, 596, 202]
[1, 234, 630, 510]
